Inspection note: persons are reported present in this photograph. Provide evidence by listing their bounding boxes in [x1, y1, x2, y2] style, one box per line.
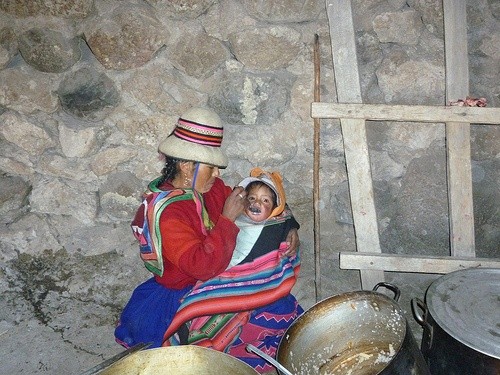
[224, 168, 286, 266]
[114, 109, 306, 375]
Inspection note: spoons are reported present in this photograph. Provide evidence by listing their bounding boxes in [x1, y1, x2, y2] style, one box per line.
[234, 185, 260, 213]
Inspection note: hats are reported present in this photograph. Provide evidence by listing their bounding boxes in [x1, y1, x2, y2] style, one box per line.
[158, 108, 228, 169]
[238, 173, 278, 207]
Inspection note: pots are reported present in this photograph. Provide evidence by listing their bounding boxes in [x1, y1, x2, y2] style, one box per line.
[275, 281, 431, 375]
[86, 345, 260, 375]
[412, 266, 500, 375]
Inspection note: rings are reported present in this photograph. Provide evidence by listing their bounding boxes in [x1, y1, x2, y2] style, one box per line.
[237, 193, 243, 198]
